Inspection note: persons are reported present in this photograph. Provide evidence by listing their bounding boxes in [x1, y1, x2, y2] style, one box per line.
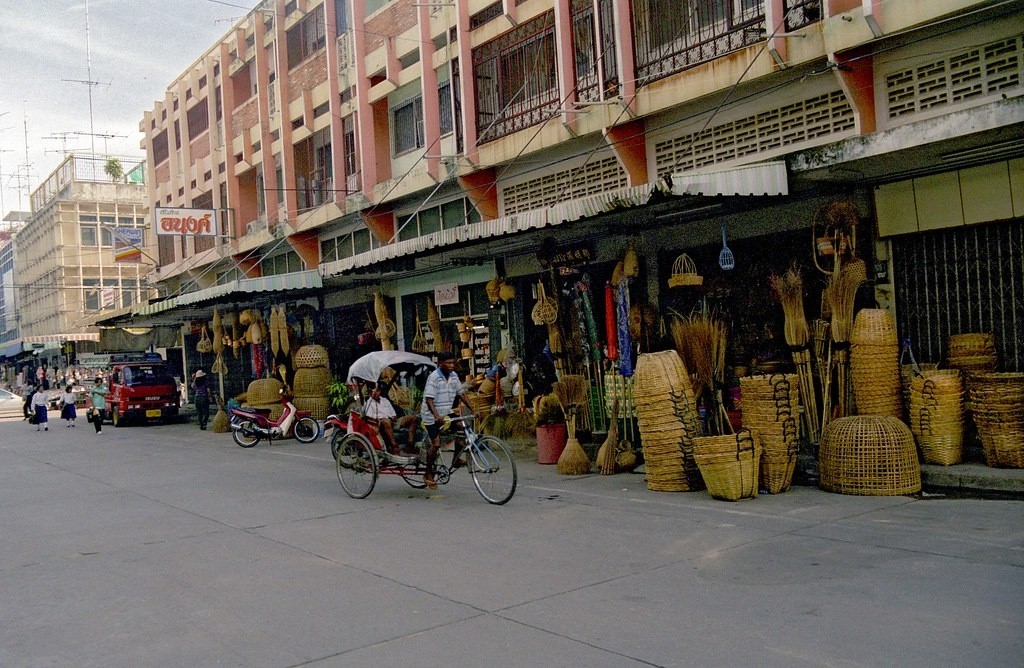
[60, 385, 77, 427]
[421, 352, 479, 490]
[31, 384, 50, 431]
[364, 381, 420, 456]
[88, 377, 107, 435]
[193, 370, 210, 431]
[23, 379, 33, 421]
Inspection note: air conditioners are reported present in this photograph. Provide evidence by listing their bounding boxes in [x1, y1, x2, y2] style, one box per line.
[245, 221, 261, 234]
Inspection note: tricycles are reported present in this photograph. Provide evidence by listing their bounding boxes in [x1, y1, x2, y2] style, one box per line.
[336, 351, 518, 505]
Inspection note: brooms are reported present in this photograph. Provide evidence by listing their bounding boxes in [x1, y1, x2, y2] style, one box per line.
[213, 393, 229, 433]
[667, 315, 737, 437]
[552, 374, 589, 439]
[505, 369, 537, 441]
[600, 395, 622, 477]
[477, 372, 515, 441]
[765, 257, 870, 446]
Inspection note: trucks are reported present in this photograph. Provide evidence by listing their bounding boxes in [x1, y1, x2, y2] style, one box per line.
[86, 362, 182, 427]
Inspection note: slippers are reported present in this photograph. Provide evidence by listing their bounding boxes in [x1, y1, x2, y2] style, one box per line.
[422, 475, 437, 490]
[452, 459, 469, 468]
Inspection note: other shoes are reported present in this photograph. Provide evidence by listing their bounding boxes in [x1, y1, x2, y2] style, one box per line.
[45, 426, 48, 431]
[67, 424, 70, 427]
[72, 424, 75, 427]
[392, 443, 401, 456]
[97, 431, 102, 435]
[401, 444, 421, 457]
[36, 428, 40, 431]
[201, 422, 208, 430]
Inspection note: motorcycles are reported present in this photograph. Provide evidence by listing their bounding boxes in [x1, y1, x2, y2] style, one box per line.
[230, 385, 320, 448]
[324, 413, 363, 470]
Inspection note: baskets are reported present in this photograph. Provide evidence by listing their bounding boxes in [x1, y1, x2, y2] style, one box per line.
[629, 307, 1024, 501]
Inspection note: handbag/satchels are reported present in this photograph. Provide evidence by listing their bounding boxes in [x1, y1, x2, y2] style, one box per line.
[93, 409, 99, 416]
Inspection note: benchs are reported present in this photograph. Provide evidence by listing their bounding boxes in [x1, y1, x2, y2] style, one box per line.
[351, 404, 423, 450]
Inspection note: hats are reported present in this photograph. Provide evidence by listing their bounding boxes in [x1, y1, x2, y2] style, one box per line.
[196, 370, 206, 377]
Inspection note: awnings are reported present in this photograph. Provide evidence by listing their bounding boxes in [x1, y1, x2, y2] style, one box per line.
[77, 269, 323, 327]
[317, 160, 788, 279]
